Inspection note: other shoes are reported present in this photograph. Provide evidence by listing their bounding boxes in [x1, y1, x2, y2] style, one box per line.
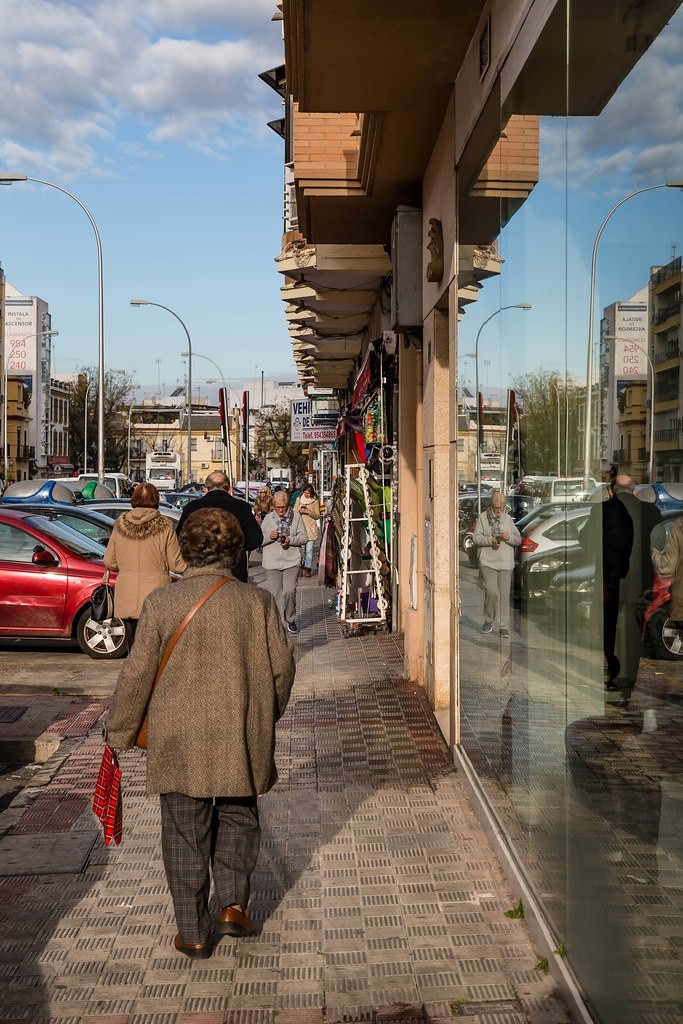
[305, 567, 311, 577]
[298, 566, 304, 576]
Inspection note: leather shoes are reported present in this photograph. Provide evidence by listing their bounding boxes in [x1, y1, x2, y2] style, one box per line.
[175, 933, 211, 958]
[220, 905, 253, 938]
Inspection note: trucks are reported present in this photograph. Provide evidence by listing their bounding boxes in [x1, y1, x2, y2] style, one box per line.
[269, 468, 294, 493]
[144, 450, 181, 494]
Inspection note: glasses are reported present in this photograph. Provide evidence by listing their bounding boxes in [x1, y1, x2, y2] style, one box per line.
[260, 491, 266, 494]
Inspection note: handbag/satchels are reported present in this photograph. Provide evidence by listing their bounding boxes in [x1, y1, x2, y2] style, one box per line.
[137, 713, 150, 750]
[91, 749, 123, 847]
[90, 568, 115, 621]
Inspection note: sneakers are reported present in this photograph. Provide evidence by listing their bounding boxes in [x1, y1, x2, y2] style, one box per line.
[482, 620, 491, 635]
[500, 629, 509, 637]
[287, 620, 297, 633]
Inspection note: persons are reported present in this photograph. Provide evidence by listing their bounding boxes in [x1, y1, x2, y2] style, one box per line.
[105, 471, 320, 654]
[472, 492, 521, 638]
[102, 507, 296, 961]
[579, 472, 683, 706]
[0, 479, 5, 504]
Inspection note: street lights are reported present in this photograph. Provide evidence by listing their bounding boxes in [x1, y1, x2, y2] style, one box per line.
[473, 302, 534, 522]
[0, 172, 106, 485]
[523, 372, 562, 480]
[575, 176, 683, 491]
[603, 335, 655, 487]
[83, 369, 125, 474]
[130, 298, 193, 486]
[3, 329, 59, 483]
[126, 390, 165, 476]
[180, 352, 234, 497]
[206, 378, 244, 481]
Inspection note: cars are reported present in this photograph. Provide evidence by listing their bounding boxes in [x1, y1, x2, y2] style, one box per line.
[460, 452, 683, 659]
[0, 507, 183, 660]
[0, 481, 288, 548]
